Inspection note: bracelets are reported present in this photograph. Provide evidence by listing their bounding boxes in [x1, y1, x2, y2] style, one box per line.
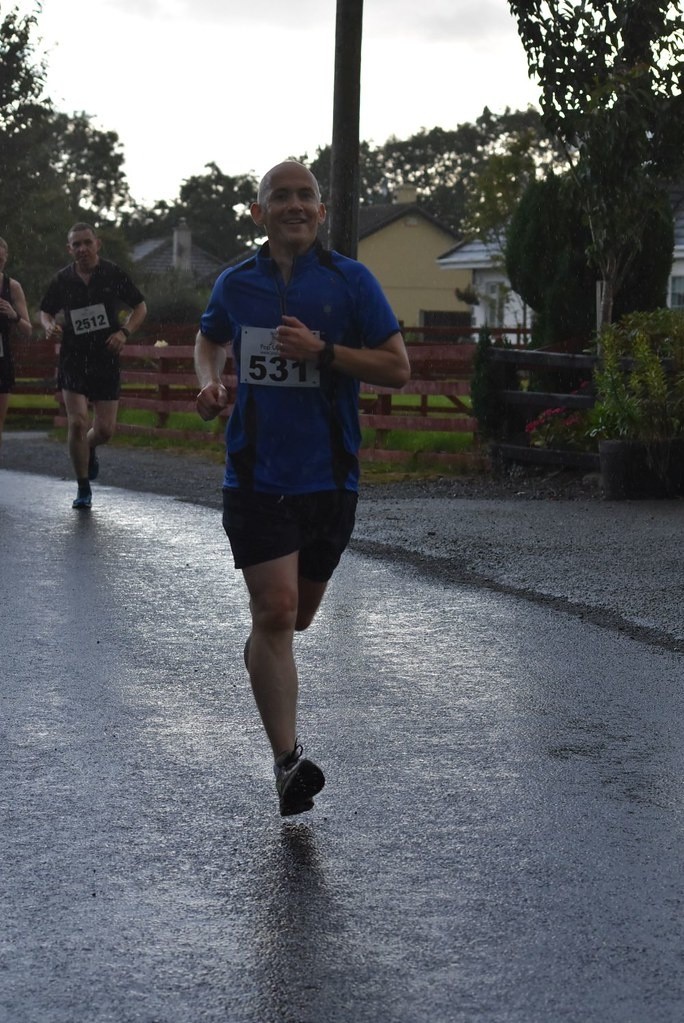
[118, 328, 130, 342]
[315, 334, 335, 370]
[7, 312, 22, 323]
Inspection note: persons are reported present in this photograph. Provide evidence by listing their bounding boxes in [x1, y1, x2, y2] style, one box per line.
[0, 237, 32, 449]
[194, 162, 411, 817]
[39, 222, 147, 509]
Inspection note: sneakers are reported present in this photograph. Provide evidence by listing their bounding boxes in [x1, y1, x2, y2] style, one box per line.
[72, 490, 92, 508]
[273, 740, 324, 818]
[88, 446, 99, 481]
